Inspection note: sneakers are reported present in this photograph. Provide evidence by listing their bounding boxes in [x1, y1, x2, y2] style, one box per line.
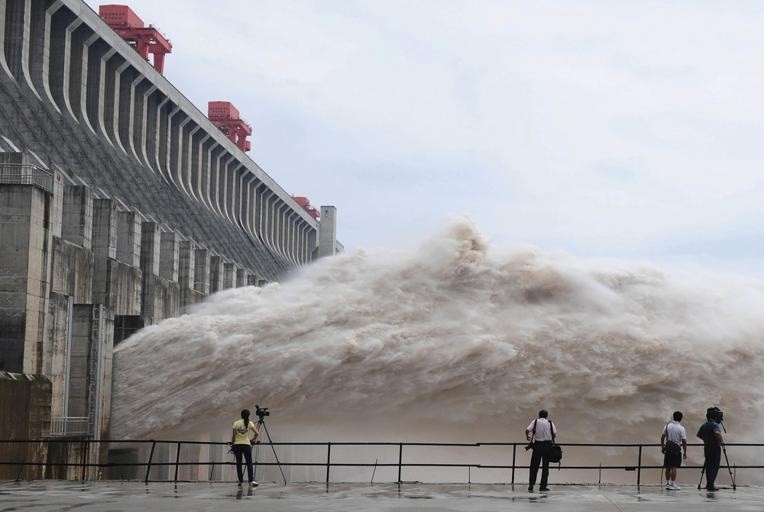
[669, 482, 680, 490]
[665, 483, 671, 489]
[249, 480, 259, 487]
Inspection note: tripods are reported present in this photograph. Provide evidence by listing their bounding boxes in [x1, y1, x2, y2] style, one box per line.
[241, 418, 287, 485]
[697, 443, 736, 490]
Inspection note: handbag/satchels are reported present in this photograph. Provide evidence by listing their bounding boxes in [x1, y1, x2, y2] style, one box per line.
[549, 444, 563, 463]
[663, 440, 682, 456]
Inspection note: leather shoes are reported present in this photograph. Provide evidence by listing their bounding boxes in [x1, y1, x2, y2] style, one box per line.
[707, 486, 719, 490]
[540, 486, 549, 490]
[528, 485, 533, 490]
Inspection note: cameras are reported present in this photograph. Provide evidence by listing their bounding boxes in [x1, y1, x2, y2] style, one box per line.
[526, 442, 534, 451]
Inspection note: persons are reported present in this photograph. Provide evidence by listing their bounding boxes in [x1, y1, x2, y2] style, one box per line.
[229, 409, 260, 489]
[695, 407, 723, 491]
[525, 409, 557, 492]
[661, 411, 688, 490]
